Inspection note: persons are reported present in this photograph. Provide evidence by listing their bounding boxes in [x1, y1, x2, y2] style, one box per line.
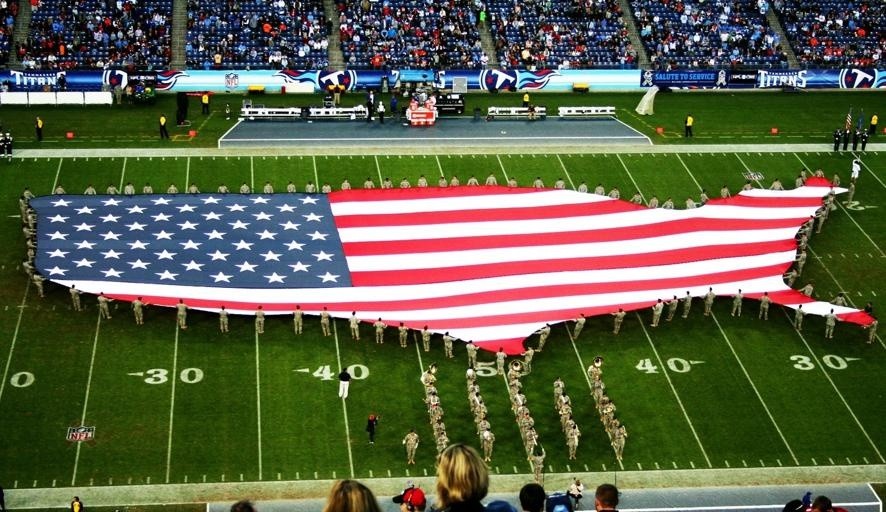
[2, 0, 886, 154]
[19, 157, 882, 510]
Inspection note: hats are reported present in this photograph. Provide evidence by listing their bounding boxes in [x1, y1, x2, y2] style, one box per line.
[391, 486, 427, 507]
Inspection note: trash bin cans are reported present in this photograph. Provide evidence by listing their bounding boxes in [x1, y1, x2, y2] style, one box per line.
[394, 111, 401, 122]
[540, 106, 546, 121]
[474, 108, 481, 120]
[298, 106, 311, 119]
